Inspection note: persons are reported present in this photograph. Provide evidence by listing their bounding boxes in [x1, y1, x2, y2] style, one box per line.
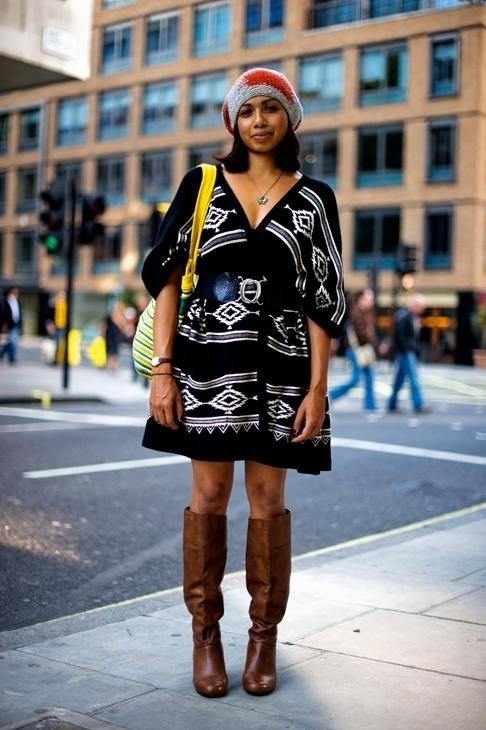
[0, 285, 22, 365]
[100, 299, 126, 371]
[379, 294, 434, 414]
[322, 285, 386, 417]
[121, 69, 348, 698]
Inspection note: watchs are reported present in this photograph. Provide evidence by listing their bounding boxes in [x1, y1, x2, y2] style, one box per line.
[151, 357, 171, 366]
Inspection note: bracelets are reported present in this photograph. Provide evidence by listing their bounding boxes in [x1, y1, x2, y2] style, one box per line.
[150, 372, 173, 376]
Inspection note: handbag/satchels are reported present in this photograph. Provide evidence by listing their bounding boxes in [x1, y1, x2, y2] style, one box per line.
[132, 273, 196, 381]
[354, 343, 377, 368]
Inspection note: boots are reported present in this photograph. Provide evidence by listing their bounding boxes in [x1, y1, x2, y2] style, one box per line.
[183, 506, 228, 698]
[242, 509, 291, 696]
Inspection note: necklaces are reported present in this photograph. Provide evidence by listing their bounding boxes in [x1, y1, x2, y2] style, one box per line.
[248, 170, 284, 204]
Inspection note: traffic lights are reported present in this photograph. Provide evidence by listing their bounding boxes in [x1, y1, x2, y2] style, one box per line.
[74, 192, 104, 250]
[399, 244, 419, 292]
[38, 177, 69, 256]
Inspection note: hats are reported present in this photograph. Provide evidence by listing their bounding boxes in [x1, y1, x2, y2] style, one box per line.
[222, 68, 303, 136]
